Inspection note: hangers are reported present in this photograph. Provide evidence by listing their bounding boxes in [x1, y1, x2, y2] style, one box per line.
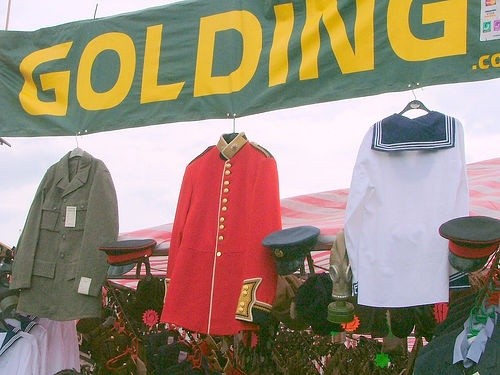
[45, 129, 105, 172]
[367, 81, 465, 138]
[186, 113, 276, 169]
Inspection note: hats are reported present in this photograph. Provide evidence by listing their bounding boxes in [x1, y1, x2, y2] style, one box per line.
[100, 238, 156, 276]
[271, 276, 310, 331]
[295, 273, 338, 336]
[439, 216, 499, 273]
[328, 230, 353, 298]
[262, 225, 320, 276]
